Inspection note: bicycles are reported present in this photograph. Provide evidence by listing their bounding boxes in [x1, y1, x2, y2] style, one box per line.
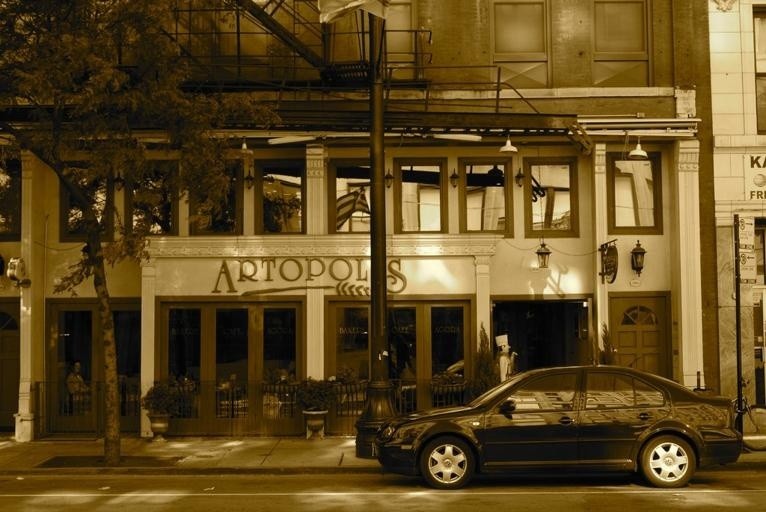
[692, 377, 766, 452]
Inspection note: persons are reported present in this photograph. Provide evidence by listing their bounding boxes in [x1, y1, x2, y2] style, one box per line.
[493, 333, 519, 383]
[65, 360, 89, 400]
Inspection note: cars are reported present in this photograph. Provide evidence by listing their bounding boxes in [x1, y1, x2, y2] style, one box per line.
[376, 363, 743, 490]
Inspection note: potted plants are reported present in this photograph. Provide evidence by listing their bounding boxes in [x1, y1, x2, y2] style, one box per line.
[142, 384, 181, 443]
[296, 376, 338, 440]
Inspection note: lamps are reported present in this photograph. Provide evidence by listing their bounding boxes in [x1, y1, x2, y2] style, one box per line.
[244, 170, 255, 189]
[631, 240, 646, 277]
[449, 168, 460, 188]
[628, 136, 648, 160]
[515, 167, 525, 187]
[537, 244, 551, 268]
[385, 169, 395, 188]
[500, 134, 518, 155]
